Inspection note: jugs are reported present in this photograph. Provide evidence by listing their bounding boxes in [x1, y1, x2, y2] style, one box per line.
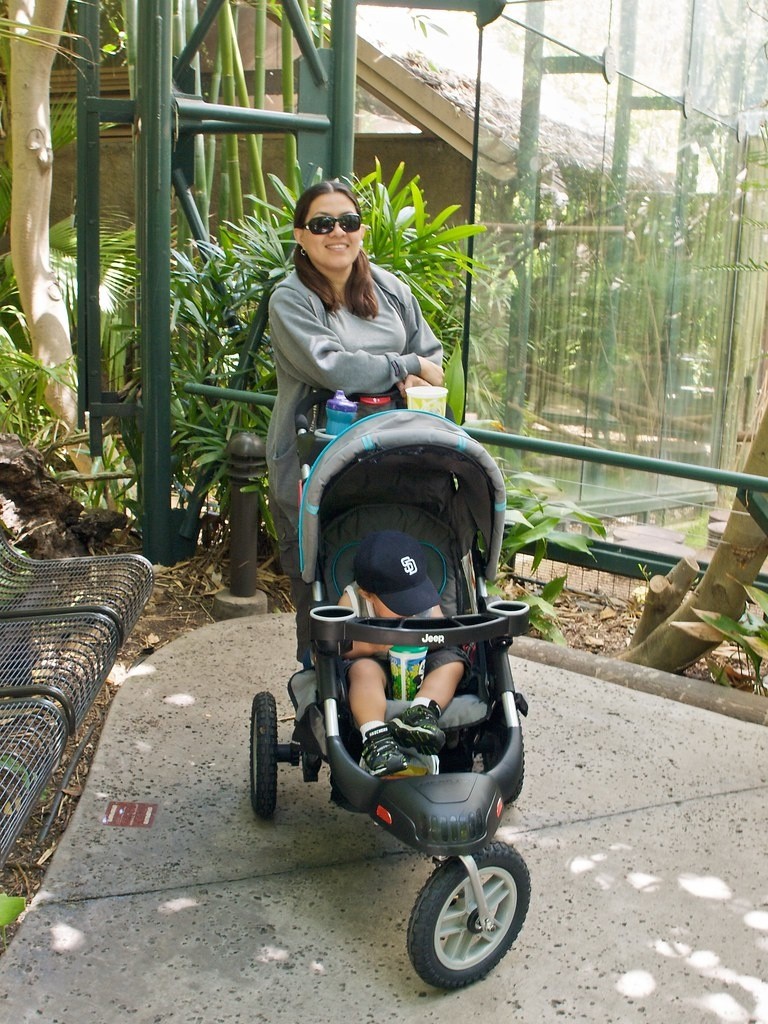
[389, 645, 428, 701]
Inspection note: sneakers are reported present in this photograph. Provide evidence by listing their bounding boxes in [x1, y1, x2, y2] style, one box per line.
[387, 699, 445, 755]
[360, 725, 409, 776]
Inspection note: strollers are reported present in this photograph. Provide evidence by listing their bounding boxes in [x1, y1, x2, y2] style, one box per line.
[247, 383, 536, 991]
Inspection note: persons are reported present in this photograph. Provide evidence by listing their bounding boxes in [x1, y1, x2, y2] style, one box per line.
[335, 526, 472, 778]
[265, 181, 445, 663]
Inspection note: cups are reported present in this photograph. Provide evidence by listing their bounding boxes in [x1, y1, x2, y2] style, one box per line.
[406, 385, 448, 418]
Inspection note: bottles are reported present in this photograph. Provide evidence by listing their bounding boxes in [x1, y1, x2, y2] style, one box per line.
[327, 390, 356, 436]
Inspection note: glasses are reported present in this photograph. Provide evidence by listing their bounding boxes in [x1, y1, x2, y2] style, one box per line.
[301, 214, 361, 235]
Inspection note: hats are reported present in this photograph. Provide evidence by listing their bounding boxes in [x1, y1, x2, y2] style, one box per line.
[354, 530, 441, 616]
